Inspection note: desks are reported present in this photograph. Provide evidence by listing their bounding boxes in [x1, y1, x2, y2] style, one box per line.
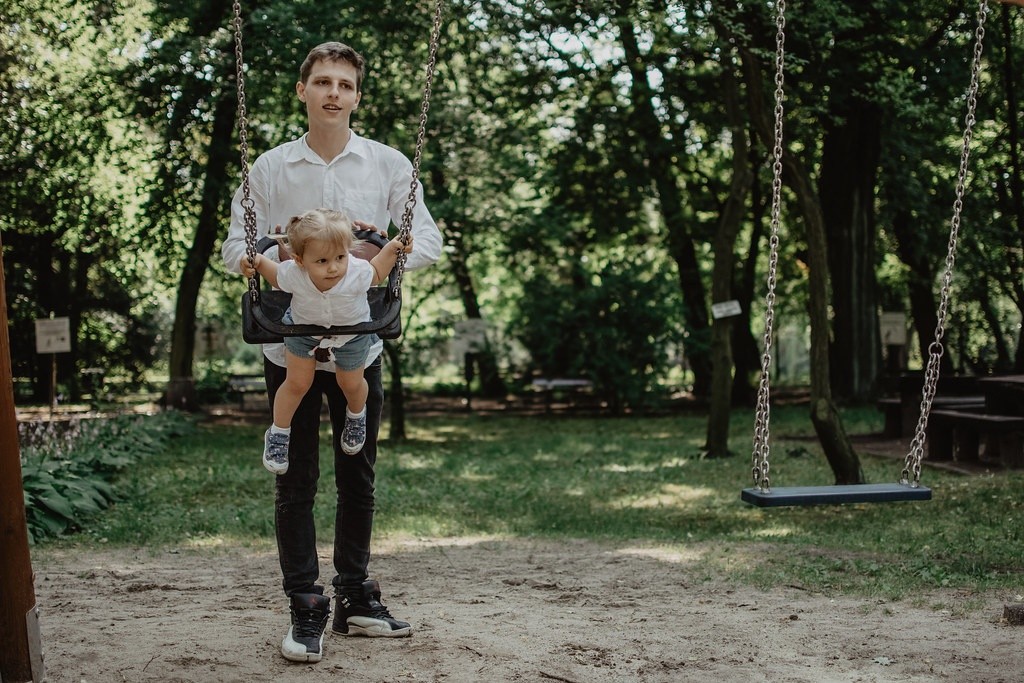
[228, 372, 266, 387]
[978, 375, 1024, 465]
[897, 374, 976, 438]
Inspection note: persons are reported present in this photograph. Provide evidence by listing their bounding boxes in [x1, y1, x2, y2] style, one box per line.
[243, 208, 414, 475]
[221, 42, 443, 662]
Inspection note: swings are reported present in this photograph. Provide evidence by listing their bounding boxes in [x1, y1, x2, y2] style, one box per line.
[233, 1, 447, 345]
[738, 0, 991, 511]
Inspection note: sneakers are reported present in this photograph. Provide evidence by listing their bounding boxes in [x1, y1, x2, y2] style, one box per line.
[340, 406, 367, 455]
[281, 593, 331, 662]
[331, 574, 413, 637]
[262, 425, 290, 475]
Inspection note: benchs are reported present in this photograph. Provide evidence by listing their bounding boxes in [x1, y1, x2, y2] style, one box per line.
[877, 398, 899, 440]
[927, 409, 1024, 463]
[236, 388, 268, 411]
[923, 397, 985, 410]
